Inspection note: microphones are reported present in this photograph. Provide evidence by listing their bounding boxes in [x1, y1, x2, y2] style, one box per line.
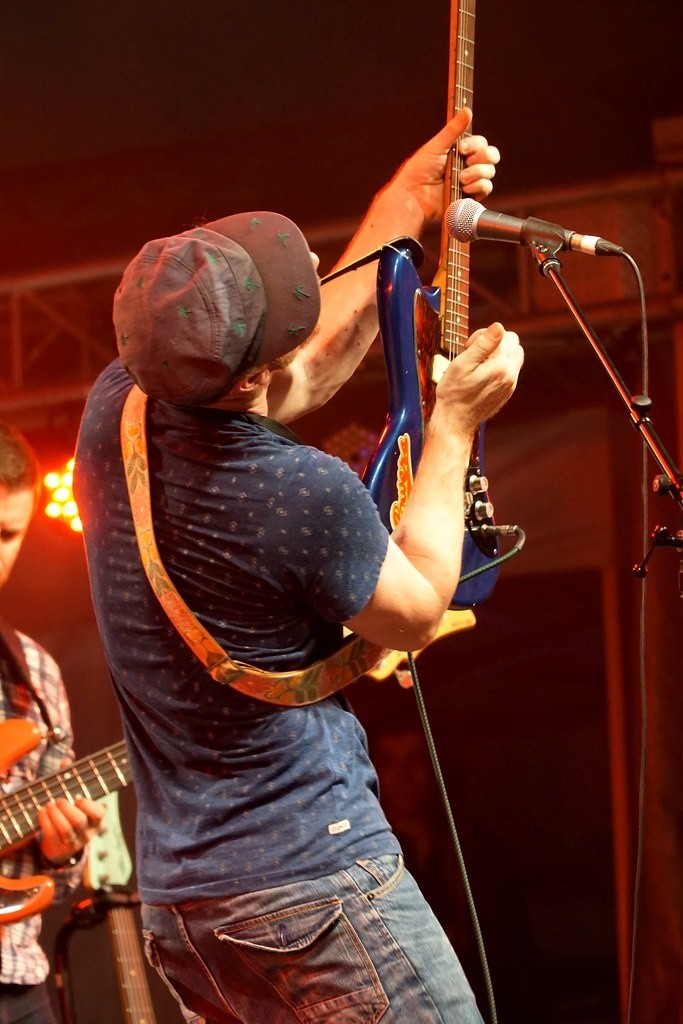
[444, 196, 624, 256]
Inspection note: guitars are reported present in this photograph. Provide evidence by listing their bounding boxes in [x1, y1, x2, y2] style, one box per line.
[0, 715, 133, 925]
[363, 0, 503, 612]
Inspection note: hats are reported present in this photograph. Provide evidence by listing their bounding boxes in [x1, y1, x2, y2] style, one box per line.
[112, 211, 320, 408]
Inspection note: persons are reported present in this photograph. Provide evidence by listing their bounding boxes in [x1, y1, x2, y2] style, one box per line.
[0, 418, 132, 1024]
[71, 107, 524, 1024]
